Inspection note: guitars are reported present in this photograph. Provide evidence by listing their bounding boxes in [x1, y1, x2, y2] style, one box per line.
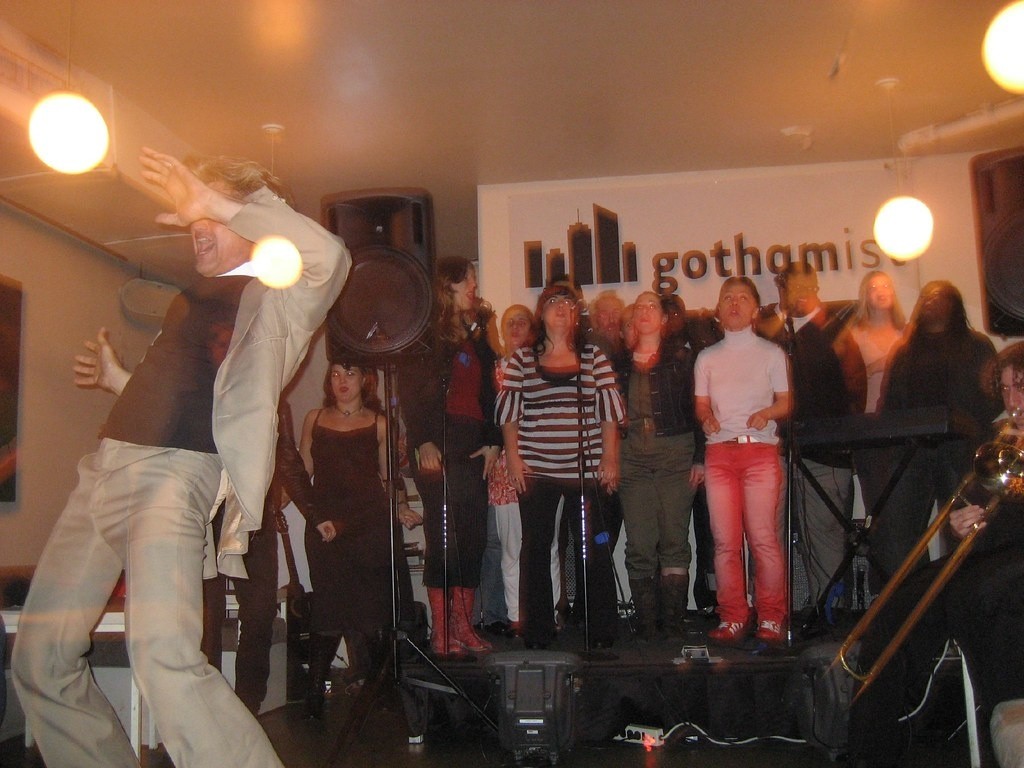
[276, 511, 314, 665]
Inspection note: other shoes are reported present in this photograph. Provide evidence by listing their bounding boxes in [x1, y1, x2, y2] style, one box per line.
[526, 640, 551, 649]
[485, 621, 517, 638]
[587, 639, 613, 649]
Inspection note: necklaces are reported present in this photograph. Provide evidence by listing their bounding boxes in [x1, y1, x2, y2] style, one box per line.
[336, 407, 362, 414]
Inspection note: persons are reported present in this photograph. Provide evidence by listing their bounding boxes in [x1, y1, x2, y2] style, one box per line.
[281, 257, 503, 680]
[11, 145, 352, 768]
[199, 392, 338, 711]
[484, 264, 863, 649]
[838, 270, 1023, 768]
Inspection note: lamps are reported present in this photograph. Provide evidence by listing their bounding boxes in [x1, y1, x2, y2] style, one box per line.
[872, 78, 936, 266]
[28, 0, 111, 175]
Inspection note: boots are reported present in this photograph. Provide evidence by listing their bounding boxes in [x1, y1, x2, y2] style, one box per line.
[628, 576, 659, 627]
[660, 574, 689, 628]
[426, 586, 466, 653]
[446, 587, 493, 652]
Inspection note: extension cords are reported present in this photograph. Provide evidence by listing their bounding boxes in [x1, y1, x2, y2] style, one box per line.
[624, 725, 664, 746]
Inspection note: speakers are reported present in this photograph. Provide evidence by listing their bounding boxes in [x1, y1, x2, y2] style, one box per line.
[969, 144, 1024, 340]
[321, 188, 440, 370]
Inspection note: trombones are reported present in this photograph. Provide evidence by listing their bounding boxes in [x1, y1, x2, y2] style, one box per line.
[816, 408, 1024, 721]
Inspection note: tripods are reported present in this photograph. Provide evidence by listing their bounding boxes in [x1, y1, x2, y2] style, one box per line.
[325, 362, 499, 767]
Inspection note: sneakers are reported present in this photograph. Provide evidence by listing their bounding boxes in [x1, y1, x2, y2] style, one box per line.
[709, 621, 754, 639]
[754, 620, 788, 640]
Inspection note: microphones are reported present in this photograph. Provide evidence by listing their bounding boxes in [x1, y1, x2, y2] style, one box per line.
[578, 298, 590, 320]
[773, 272, 789, 286]
[467, 301, 492, 338]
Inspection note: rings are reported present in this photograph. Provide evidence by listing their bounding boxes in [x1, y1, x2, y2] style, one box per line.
[515, 477, 518, 480]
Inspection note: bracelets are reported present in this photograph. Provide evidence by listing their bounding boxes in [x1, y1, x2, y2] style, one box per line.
[398, 500, 408, 506]
